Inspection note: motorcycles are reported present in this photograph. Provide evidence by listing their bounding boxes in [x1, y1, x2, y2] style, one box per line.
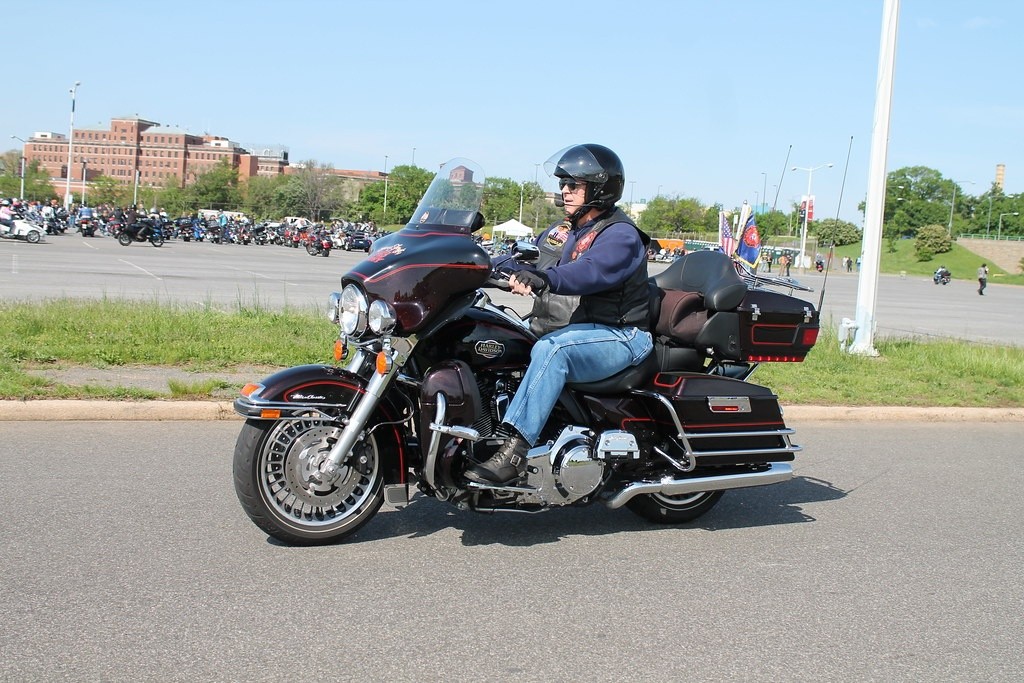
[0, 193, 392, 259]
[470, 233, 542, 258]
[933, 268, 951, 285]
[229, 132, 854, 547]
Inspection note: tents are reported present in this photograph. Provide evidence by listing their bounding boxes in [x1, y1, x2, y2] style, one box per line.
[492, 219, 533, 242]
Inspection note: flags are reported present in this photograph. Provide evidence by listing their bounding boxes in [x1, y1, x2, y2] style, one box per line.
[721, 214, 735, 257]
[731, 206, 762, 268]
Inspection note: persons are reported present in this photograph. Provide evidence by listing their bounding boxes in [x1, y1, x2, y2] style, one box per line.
[648, 246, 685, 258]
[68, 203, 86, 229]
[0, 199, 21, 232]
[280, 216, 378, 231]
[463, 144, 654, 487]
[816, 260, 823, 269]
[937, 265, 948, 285]
[762, 254, 791, 277]
[530, 214, 581, 338]
[99, 203, 167, 239]
[483, 232, 515, 251]
[36, 201, 67, 228]
[198, 209, 259, 243]
[977, 264, 988, 295]
[843, 255, 861, 272]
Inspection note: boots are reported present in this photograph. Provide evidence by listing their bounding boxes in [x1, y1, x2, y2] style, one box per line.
[464, 437, 529, 487]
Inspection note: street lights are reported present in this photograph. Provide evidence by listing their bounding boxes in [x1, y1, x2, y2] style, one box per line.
[948, 179, 976, 236]
[10, 134, 26, 199]
[773, 184, 778, 212]
[986, 195, 1014, 235]
[761, 172, 767, 215]
[63, 79, 81, 212]
[629, 181, 636, 218]
[998, 213, 1019, 241]
[792, 163, 834, 270]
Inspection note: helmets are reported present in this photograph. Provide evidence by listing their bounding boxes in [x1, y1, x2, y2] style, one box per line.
[553, 143, 625, 211]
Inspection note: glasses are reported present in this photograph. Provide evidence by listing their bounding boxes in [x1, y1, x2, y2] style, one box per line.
[559, 178, 586, 190]
